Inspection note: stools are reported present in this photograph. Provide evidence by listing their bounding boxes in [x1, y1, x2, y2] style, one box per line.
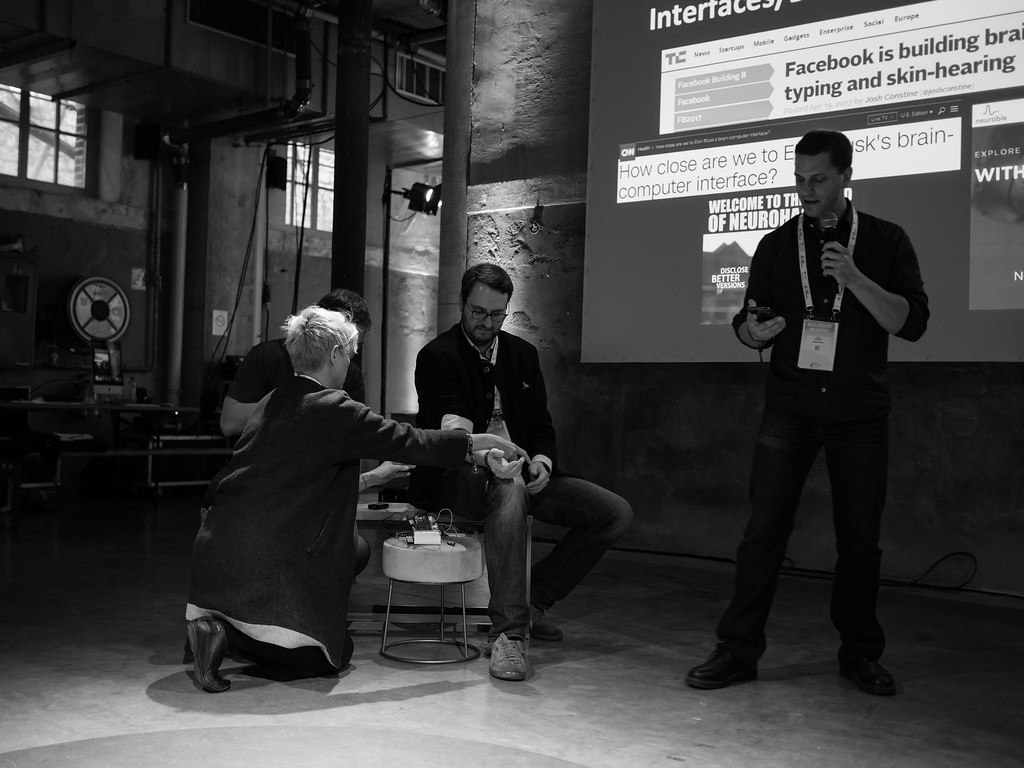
[379, 532, 486, 665]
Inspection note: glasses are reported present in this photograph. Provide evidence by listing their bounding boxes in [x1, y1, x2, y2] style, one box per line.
[465, 302, 510, 322]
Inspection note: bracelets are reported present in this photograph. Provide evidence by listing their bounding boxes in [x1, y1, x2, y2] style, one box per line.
[484, 452, 491, 472]
[361, 473, 372, 488]
[746, 326, 772, 345]
[467, 434, 474, 456]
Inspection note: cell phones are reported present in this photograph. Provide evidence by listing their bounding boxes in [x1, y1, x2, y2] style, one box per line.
[368, 503, 389, 510]
[746, 306, 778, 322]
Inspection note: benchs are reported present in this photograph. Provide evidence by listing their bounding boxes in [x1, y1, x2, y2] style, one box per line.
[351, 498, 534, 626]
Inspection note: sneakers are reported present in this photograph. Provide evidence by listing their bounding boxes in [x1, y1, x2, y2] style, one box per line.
[529, 606, 563, 640]
[488, 632, 527, 680]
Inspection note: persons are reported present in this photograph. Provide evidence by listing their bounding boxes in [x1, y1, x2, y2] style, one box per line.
[686, 130, 930, 697]
[408, 263, 634, 681]
[182, 288, 533, 693]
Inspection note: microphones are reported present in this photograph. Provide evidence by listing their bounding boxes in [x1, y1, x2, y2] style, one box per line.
[820, 212, 840, 295]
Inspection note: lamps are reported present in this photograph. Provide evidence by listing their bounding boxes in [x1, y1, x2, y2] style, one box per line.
[402, 183, 435, 203]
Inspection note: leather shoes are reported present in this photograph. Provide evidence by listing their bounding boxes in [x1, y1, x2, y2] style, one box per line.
[685, 642, 759, 689]
[836, 642, 902, 694]
[187, 619, 232, 693]
